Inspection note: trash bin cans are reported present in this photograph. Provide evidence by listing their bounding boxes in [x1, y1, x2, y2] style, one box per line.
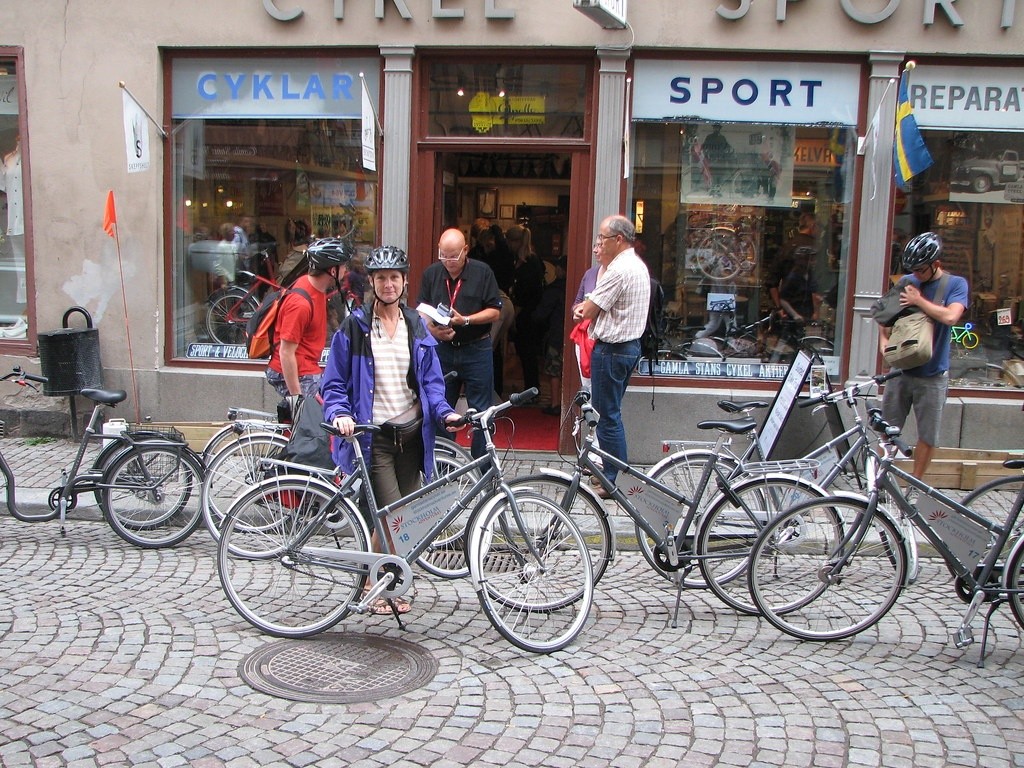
[37, 326, 105, 396]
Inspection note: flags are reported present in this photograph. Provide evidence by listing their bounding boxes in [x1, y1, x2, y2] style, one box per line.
[895, 70, 933, 189]
[123, 87, 152, 174]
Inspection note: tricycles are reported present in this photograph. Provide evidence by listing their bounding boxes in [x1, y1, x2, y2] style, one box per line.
[1, 366, 211, 549]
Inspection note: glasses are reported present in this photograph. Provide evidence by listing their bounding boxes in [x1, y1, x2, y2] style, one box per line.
[599, 234, 621, 243]
[439, 246, 467, 264]
[912, 264, 935, 276]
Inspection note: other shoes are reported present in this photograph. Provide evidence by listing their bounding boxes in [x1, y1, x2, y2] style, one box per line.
[876, 490, 890, 502]
[590, 476, 600, 483]
[906, 486, 920, 506]
[593, 486, 610, 499]
[547, 403, 561, 416]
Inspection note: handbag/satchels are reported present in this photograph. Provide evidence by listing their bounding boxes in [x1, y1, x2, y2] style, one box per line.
[374, 399, 423, 454]
[568, 319, 595, 379]
[275, 395, 336, 498]
[870, 279, 937, 371]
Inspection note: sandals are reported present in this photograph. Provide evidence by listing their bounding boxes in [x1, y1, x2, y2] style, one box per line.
[360, 596, 412, 614]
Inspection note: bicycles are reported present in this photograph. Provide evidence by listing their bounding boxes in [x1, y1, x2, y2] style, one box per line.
[202, 224, 362, 347]
[685, 221, 757, 279]
[461, 386, 847, 619]
[640, 298, 835, 373]
[680, 144, 763, 199]
[200, 369, 497, 579]
[946, 342, 1022, 388]
[634, 368, 921, 589]
[745, 367, 1024, 669]
[217, 387, 593, 654]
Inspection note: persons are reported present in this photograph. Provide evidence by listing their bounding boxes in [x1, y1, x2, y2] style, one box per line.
[267, 237, 361, 420]
[870, 231, 967, 525]
[585, 214, 651, 497]
[572, 235, 605, 475]
[3, 137, 27, 324]
[467, 219, 565, 417]
[194, 213, 365, 316]
[418, 228, 502, 485]
[320, 246, 468, 614]
[632, 212, 845, 364]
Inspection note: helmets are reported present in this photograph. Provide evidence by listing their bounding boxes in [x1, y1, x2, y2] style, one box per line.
[903, 230, 943, 271]
[305, 238, 353, 274]
[365, 244, 408, 270]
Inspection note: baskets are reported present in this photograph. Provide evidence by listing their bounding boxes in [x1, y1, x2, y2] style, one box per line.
[124, 424, 183, 482]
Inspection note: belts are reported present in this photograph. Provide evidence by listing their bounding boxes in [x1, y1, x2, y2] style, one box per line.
[450, 332, 491, 346]
